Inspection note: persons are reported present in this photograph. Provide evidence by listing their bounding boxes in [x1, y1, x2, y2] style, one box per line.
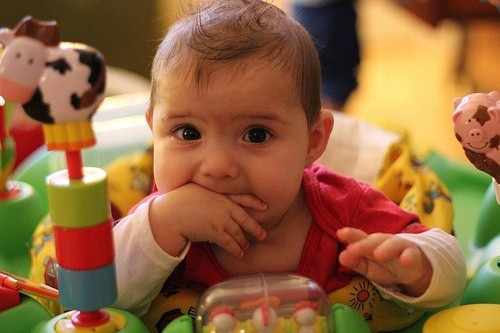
[103, 0, 467, 318]
[290, 0, 362, 112]
